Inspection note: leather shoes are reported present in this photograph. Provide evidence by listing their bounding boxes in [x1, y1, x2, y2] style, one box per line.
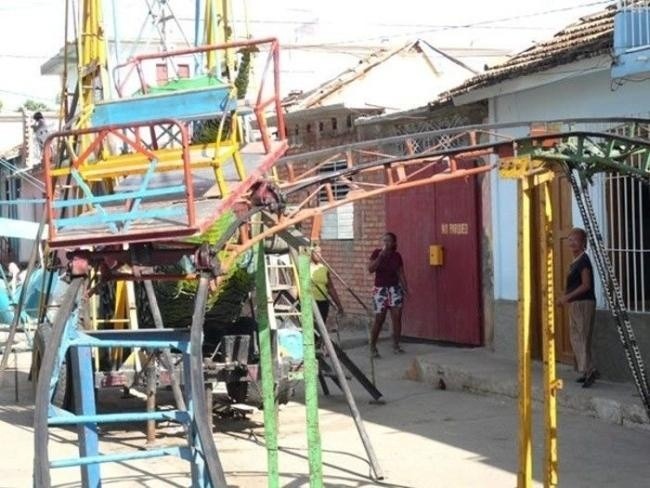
[575, 367, 601, 388]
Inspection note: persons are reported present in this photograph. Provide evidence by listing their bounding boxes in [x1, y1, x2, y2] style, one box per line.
[309, 246, 343, 341]
[553, 226, 601, 391]
[366, 231, 411, 359]
